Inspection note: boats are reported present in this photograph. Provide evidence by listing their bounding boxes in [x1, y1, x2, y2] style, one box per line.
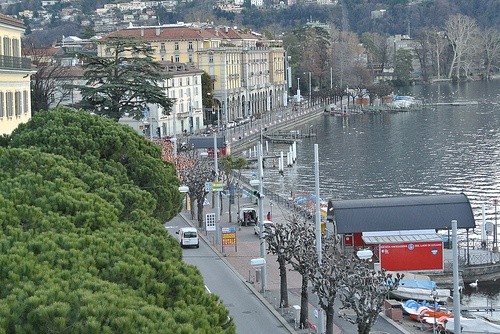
[381, 271, 500, 334]
[294, 193, 328, 219]
[392, 96, 418, 109]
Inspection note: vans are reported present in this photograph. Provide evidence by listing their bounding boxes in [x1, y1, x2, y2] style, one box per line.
[175, 227, 199, 248]
[237, 208, 257, 226]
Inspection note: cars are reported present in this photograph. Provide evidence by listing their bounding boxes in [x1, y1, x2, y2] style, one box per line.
[254, 221, 276, 239]
[292, 95, 304, 105]
[192, 112, 262, 136]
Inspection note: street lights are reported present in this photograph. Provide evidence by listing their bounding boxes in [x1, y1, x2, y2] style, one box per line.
[297, 77, 300, 103]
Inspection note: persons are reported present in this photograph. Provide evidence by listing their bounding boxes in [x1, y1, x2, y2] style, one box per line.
[245, 212, 251, 226]
[267, 212, 271, 221]
